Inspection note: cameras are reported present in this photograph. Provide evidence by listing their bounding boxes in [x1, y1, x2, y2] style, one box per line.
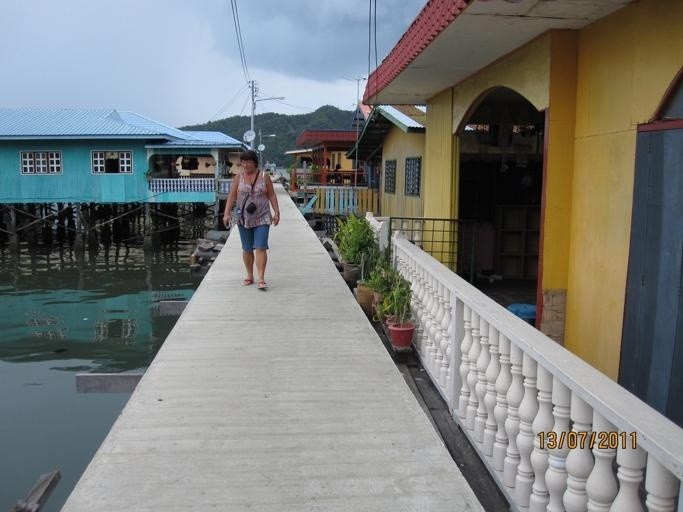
[246, 202, 257, 215]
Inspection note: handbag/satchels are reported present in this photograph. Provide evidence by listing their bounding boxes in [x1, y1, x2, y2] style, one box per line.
[234, 206, 245, 226]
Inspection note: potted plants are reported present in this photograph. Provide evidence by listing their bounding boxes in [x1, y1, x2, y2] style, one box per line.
[333, 214, 415, 351]
[296, 179, 304, 197]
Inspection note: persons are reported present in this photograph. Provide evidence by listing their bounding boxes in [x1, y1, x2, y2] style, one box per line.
[223, 150, 280, 289]
[334, 164, 341, 172]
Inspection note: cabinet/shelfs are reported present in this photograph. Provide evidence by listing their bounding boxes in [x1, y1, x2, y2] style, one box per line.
[495, 205, 540, 281]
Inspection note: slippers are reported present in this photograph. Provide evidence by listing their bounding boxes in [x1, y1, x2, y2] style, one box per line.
[257, 280, 268, 289]
[241, 280, 253, 286]
